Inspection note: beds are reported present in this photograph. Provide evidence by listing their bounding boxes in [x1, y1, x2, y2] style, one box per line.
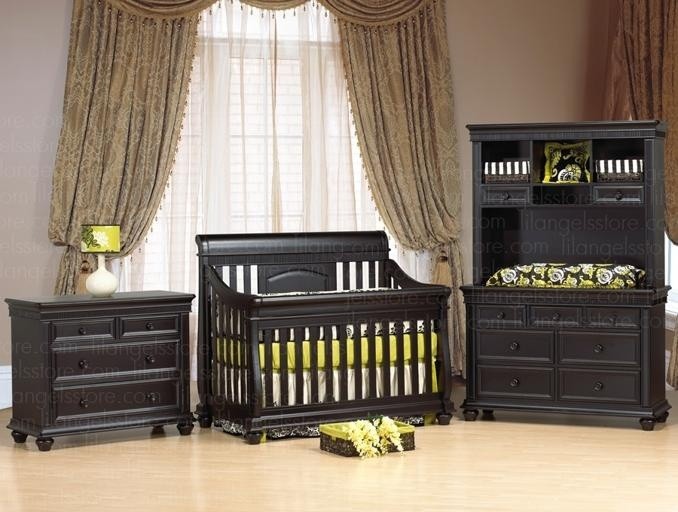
[193, 232, 454, 442]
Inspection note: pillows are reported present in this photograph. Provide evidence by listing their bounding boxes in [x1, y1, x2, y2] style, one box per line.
[540, 140, 593, 183]
[482, 263, 648, 290]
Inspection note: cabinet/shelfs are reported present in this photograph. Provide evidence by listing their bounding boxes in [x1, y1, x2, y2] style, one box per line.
[461, 120, 672, 288]
[2, 288, 195, 452]
[459, 285, 673, 431]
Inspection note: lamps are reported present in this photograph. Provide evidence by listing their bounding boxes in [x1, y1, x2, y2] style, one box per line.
[78, 221, 129, 296]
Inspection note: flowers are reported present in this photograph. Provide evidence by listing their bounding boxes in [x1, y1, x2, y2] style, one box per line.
[343, 413, 405, 457]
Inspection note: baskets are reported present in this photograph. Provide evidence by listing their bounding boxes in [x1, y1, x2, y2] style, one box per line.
[318, 416, 416, 458]
